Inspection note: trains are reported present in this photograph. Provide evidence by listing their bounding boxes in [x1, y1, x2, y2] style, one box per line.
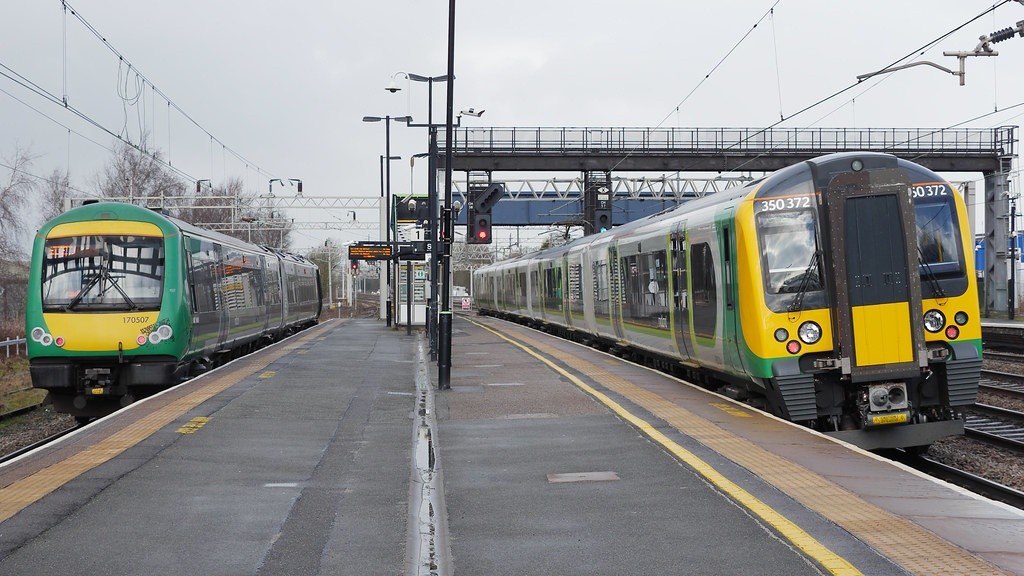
[473, 151, 983, 458]
[26, 200, 323, 426]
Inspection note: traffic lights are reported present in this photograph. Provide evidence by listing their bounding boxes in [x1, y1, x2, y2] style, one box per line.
[594, 210, 612, 233]
[351, 260, 358, 269]
[475, 214, 490, 243]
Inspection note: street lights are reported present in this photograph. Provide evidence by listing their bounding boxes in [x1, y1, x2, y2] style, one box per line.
[362, 115, 413, 327]
[406, 72, 456, 361]
[380, 155, 402, 319]
